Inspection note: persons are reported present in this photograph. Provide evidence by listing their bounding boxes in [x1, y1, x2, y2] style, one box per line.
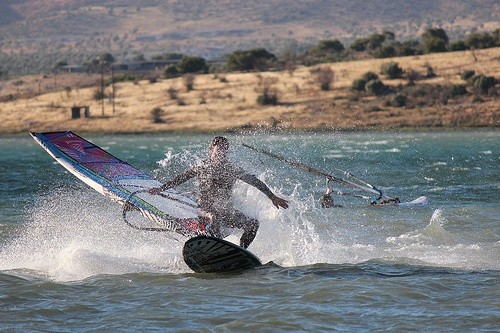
[148, 136, 288, 250]
